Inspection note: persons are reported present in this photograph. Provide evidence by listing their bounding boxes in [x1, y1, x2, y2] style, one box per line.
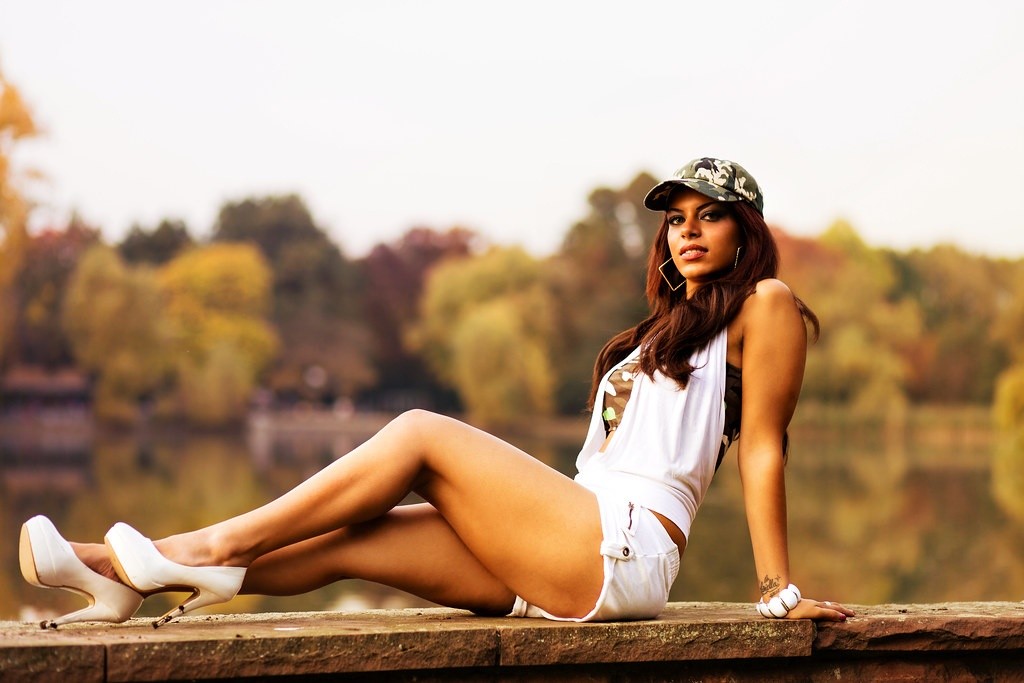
[18, 157, 853, 630]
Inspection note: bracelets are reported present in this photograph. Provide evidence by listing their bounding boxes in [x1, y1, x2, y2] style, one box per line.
[756, 584, 802, 619]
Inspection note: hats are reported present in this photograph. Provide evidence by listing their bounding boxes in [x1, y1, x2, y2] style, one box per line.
[643, 157, 764, 221]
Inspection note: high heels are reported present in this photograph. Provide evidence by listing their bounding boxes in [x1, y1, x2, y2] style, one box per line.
[103, 521, 247, 630]
[19, 515, 145, 630]
